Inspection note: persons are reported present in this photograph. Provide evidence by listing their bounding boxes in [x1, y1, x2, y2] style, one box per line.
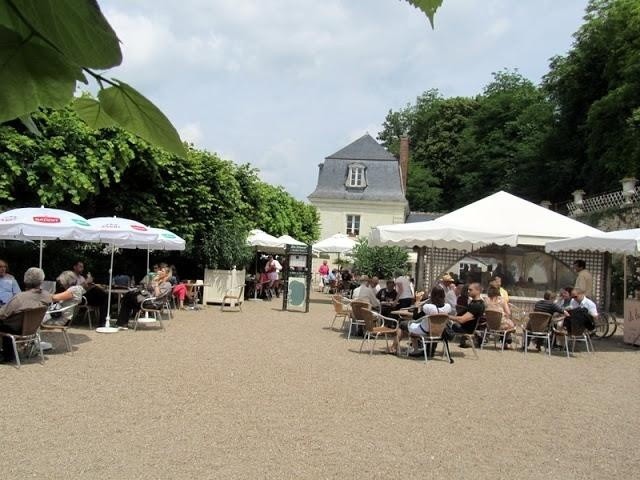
[41, 271, 84, 327]
[0, 260, 21, 304]
[72, 261, 199, 330]
[265, 255, 283, 298]
[318, 260, 600, 358]
[0, 267, 52, 364]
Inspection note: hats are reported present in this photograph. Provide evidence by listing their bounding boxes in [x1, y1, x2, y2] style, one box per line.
[267, 255, 272, 260]
[360, 275, 370, 281]
[440, 275, 455, 281]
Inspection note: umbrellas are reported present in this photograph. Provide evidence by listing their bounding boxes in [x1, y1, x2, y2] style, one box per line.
[368, 220, 518, 292]
[247, 229, 285, 273]
[118, 225, 186, 286]
[87, 215, 159, 328]
[545, 229, 640, 300]
[0, 205, 101, 269]
[258, 234, 307, 254]
[312, 231, 357, 280]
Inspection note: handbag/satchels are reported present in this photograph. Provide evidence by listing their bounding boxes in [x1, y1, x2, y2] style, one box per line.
[441, 328, 455, 341]
[51, 304, 62, 318]
[461, 334, 483, 348]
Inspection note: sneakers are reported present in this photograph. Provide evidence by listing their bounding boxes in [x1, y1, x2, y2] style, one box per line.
[115, 325, 129, 329]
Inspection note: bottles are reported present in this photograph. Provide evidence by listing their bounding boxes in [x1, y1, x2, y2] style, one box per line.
[130, 275, 135, 287]
[87, 271, 91, 278]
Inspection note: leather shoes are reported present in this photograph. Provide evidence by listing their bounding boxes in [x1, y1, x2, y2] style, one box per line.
[409, 352, 420, 357]
[363, 334, 375, 339]
[420, 350, 435, 357]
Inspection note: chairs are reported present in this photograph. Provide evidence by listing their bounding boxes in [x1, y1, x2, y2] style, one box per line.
[132, 280, 213, 334]
[329, 279, 603, 358]
[0, 306, 47, 368]
[29, 302, 80, 363]
[70, 295, 98, 331]
[219, 283, 247, 312]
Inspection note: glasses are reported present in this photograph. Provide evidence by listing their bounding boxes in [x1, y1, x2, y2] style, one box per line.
[571, 296, 578, 298]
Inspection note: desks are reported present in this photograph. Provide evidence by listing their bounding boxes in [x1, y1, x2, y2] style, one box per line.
[104, 288, 136, 325]
